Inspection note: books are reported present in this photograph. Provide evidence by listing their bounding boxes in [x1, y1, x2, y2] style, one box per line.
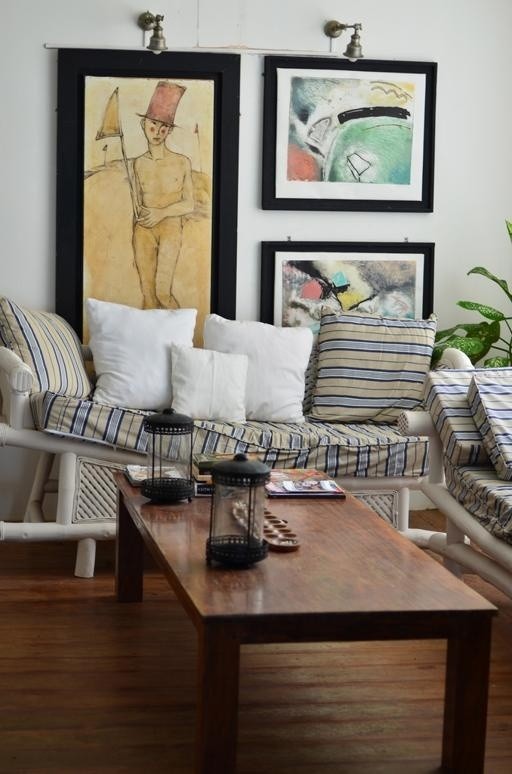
[188, 451, 262, 498]
[262, 466, 348, 504]
[123, 459, 187, 488]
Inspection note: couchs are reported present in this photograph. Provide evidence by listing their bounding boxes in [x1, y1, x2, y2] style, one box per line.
[396, 365, 510, 599]
[0, 346, 481, 576]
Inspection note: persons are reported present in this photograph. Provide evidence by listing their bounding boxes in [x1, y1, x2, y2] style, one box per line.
[129, 114, 198, 307]
